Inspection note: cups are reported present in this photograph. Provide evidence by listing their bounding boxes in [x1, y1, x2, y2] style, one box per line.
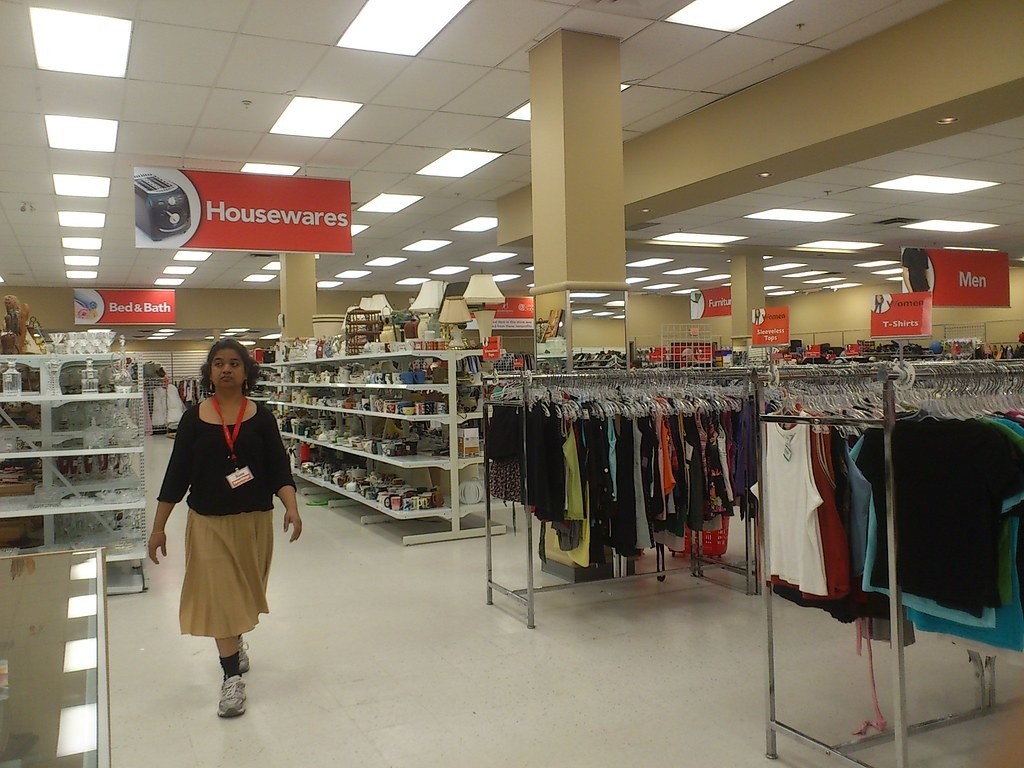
[292, 389, 448, 415]
[372, 372, 426, 384]
[360, 479, 437, 511]
[337, 430, 418, 457]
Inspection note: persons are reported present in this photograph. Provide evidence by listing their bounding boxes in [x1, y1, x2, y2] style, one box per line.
[147, 337, 303, 718]
[2, 293, 20, 335]
[754, 309, 761, 325]
[873, 293, 884, 313]
[902, 248, 933, 292]
[681, 348, 699, 367]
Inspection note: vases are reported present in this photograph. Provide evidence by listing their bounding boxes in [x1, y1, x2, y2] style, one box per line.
[418, 312, 434, 339]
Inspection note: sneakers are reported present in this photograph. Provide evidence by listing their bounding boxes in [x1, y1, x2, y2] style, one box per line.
[239, 642, 250, 673]
[217, 675, 247, 717]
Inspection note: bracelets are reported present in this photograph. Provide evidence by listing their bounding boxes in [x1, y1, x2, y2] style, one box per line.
[150, 530, 165, 536]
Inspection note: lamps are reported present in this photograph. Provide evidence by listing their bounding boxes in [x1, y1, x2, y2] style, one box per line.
[358, 297, 372, 326]
[464, 269, 507, 344]
[340, 306, 363, 334]
[438, 297, 471, 347]
[408, 281, 448, 335]
[439, 282, 485, 326]
[371, 294, 394, 340]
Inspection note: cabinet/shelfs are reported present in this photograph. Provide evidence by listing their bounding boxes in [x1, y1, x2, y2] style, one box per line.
[0, 296, 149, 596]
[245, 338, 508, 545]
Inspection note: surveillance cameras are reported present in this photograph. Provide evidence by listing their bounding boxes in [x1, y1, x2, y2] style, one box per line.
[20, 203, 26, 211]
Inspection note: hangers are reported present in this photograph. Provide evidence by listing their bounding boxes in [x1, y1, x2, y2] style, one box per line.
[492, 359, 1024, 427]
[575, 347, 616, 358]
[180, 375, 203, 382]
